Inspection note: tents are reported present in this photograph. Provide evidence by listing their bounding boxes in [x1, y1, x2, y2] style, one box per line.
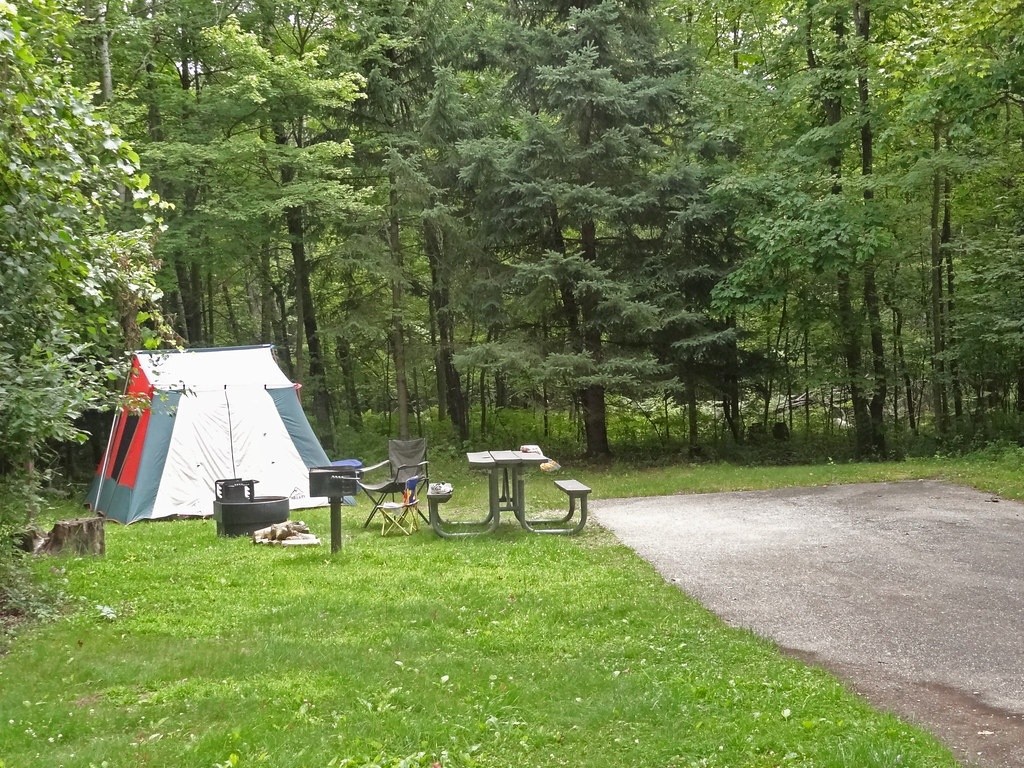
[82, 345, 356, 525]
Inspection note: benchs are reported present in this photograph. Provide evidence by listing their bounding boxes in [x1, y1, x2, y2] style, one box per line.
[549, 480, 591, 535]
[426, 483, 466, 539]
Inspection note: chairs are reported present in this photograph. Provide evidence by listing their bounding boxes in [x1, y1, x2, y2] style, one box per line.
[356, 438, 430, 529]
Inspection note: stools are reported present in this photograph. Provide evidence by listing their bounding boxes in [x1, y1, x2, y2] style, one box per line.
[377, 500, 419, 536]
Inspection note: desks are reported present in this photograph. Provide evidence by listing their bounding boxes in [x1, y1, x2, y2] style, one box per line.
[466, 450, 548, 537]
